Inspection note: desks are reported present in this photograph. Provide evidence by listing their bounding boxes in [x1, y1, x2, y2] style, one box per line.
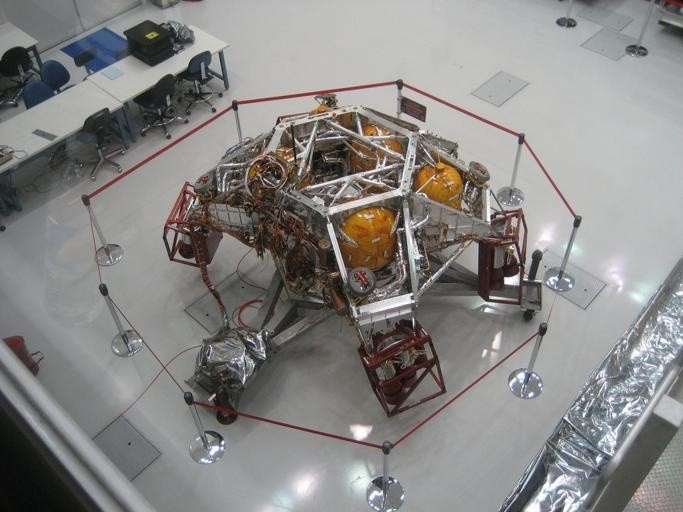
[86, 20, 231, 143]
[1, 78, 123, 212]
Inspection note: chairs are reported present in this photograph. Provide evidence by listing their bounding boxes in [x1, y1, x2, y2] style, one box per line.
[76, 107, 126, 182]
[2, 46, 38, 109]
[178, 49, 224, 113]
[20, 79, 62, 165]
[73, 48, 100, 83]
[132, 72, 188, 140]
[39, 58, 76, 95]
